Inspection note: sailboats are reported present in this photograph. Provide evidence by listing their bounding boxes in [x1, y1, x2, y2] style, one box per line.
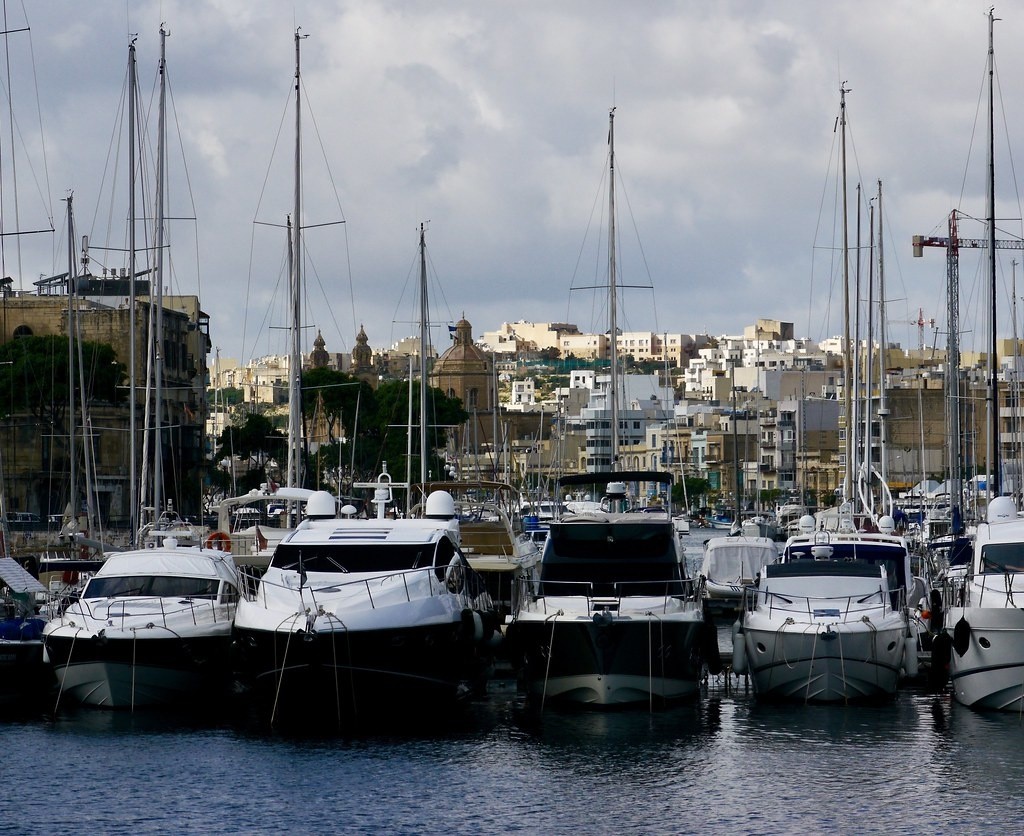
[0, 1, 1024, 716]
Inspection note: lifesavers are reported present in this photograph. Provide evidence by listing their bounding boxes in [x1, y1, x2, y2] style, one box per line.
[206, 531, 232, 552]
